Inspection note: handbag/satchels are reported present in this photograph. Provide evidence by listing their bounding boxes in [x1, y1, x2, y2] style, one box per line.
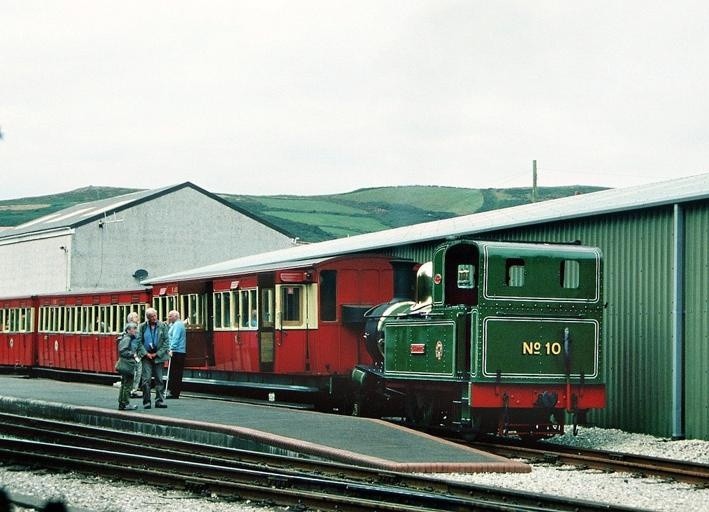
[115, 357, 136, 377]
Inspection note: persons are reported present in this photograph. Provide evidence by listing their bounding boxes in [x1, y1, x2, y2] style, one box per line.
[114, 307, 187, 410]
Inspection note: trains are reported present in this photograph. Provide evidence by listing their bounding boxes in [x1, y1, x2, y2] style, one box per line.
[1, 239, 607, 442]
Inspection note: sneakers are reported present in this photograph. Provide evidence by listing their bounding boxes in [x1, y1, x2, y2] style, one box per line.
[119, 390, 179, 410]
